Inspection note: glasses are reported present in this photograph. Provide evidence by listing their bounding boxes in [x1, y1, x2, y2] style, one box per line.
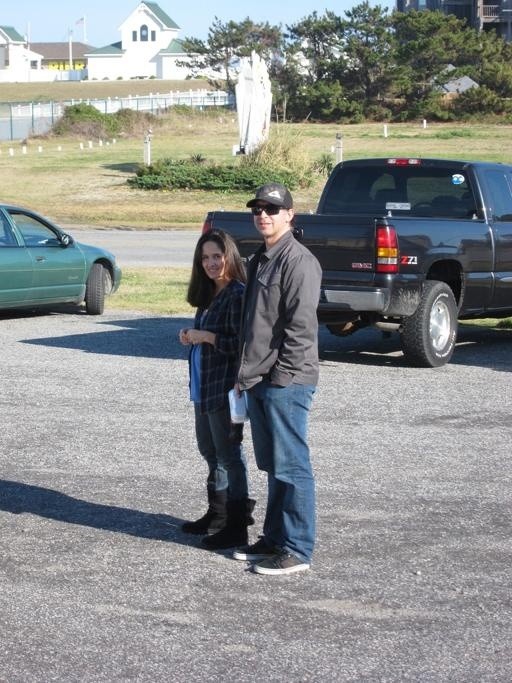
[252, 205, 283, 215]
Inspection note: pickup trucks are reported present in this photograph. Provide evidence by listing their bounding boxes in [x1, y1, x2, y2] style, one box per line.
[203, 154, 512, 366]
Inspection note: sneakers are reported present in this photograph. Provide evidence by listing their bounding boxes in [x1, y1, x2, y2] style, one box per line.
[253, 549, 310, 575]
[232, 536, 282, 560]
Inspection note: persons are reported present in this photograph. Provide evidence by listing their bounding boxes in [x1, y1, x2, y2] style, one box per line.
[233, 184, 323, 575]
[179, 229, 247, 549]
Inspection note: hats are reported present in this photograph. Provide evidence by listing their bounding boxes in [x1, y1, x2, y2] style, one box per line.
[247, 184, 292, 208]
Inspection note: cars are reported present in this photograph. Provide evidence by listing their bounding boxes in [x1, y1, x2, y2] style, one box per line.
[0, 203, 124, 317]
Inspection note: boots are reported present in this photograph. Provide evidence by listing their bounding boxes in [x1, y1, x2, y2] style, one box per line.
[202, 499, 256, 548]
[182, 491, 229, 534]
